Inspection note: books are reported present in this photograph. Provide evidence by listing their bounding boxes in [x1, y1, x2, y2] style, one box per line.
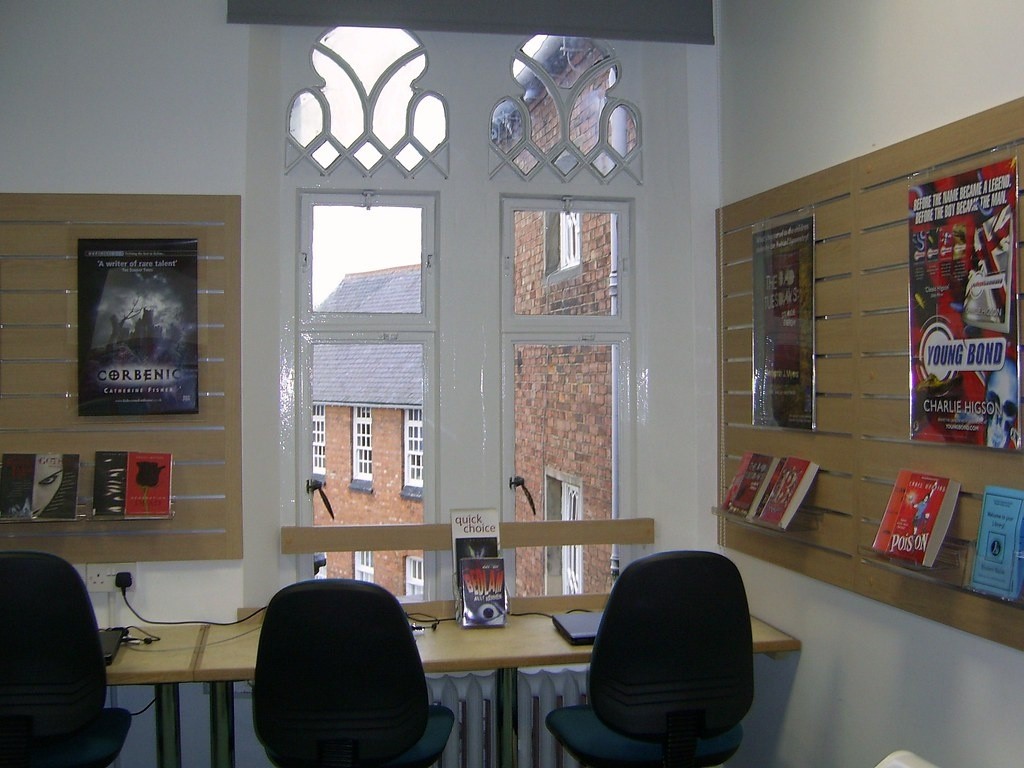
[449, 506, 508, 627]
[91, 450, 128, 517]
[883, 472, 962, 568]
[124, 453, 172, 516]
[966, 486, 1024, 600]
[0, 453, 79, 520]
[720, 450, 820, 529]
[871, 470, 912, 555]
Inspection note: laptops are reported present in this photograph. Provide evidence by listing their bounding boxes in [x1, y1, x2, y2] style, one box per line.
[552, 612, 603, 646]
[99, 630, 122, 666]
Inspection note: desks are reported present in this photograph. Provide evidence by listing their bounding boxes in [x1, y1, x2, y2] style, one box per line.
[96, 594, 801, 768]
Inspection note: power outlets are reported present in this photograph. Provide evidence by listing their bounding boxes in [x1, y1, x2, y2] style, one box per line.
[86, 562, 136, 591]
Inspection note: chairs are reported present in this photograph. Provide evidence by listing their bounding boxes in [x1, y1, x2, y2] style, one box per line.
[0, 550, 132, 768]
[253, 579, 455, 768]
[545, 550, 755, 768]
